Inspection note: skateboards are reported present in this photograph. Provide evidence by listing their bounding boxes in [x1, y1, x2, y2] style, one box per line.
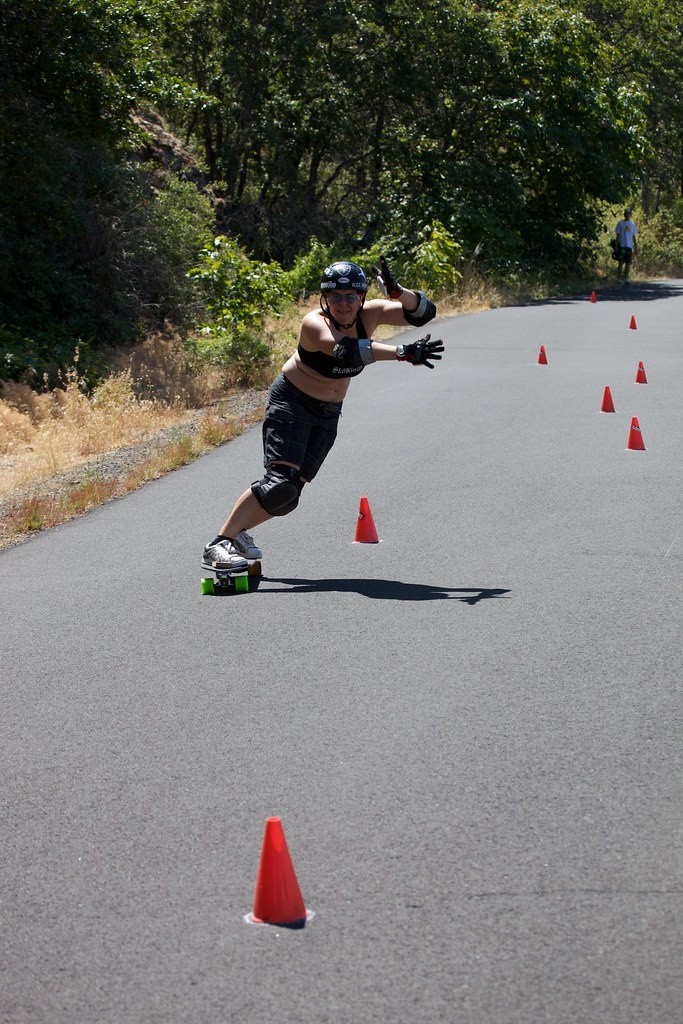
[201, 558, 257, 595]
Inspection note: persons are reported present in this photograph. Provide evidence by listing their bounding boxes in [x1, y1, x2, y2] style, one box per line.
[617, 208, 637, 279]
[200, 255, 445, 572]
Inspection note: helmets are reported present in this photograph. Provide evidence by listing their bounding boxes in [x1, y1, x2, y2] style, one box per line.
[320, 261, 368, 292]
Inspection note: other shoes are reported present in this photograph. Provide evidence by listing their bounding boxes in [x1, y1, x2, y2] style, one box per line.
[624, 279, 631, 285]
[617, 280, 623, 284]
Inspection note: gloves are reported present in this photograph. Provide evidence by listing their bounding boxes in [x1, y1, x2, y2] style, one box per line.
[371, 255, 404, 299]
[395, 333, 445, 370]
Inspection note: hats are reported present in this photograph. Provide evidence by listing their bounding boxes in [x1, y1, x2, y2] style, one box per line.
[624, 208, 632, 214]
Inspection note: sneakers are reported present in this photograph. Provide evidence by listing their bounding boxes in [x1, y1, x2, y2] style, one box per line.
[201, 539, 249, 572]
[233, 531, 263, 559]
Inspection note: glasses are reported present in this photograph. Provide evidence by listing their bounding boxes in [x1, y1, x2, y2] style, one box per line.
[329, 292, 360, 304]
